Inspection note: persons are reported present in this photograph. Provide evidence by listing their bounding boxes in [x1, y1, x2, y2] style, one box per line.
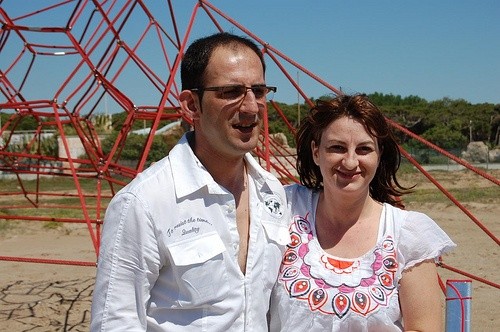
[269, 93, 458, 332]
[88, 31, 292, 332]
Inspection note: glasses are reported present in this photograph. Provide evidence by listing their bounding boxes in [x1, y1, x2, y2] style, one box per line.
[188, 85, 277, 101]
